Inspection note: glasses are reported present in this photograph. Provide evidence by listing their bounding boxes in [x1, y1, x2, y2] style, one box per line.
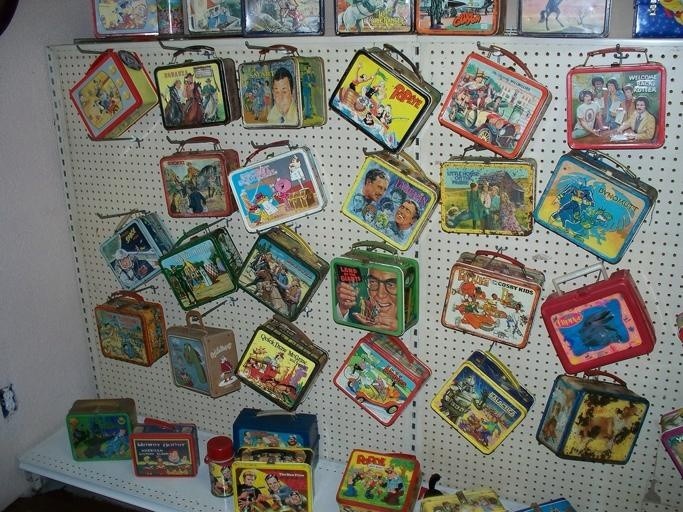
[364, 276, 398, 296]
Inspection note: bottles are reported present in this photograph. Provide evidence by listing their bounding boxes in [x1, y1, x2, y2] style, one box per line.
[202, 435, 233, 498]
